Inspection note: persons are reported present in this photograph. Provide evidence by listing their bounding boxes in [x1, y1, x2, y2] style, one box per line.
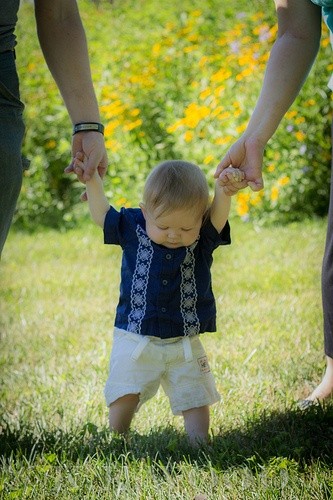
[74, 156, 244, 444]
[0, 0, 108, 261]
[212, 0, 332, 403]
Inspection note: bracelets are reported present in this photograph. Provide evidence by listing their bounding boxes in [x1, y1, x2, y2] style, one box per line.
[74, 121, 109, 137]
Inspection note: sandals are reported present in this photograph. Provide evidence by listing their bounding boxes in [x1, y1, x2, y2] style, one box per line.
[294, 397, 318, 412]
